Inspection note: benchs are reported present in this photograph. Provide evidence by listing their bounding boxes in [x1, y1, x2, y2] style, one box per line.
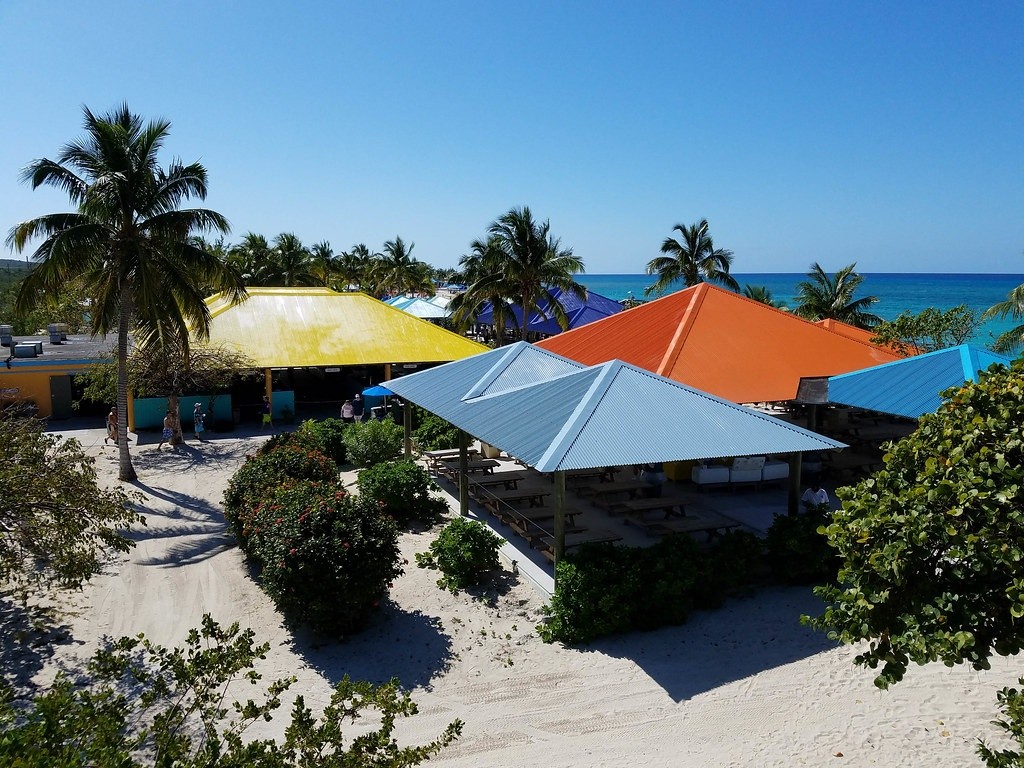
[420, 449, 741, 564]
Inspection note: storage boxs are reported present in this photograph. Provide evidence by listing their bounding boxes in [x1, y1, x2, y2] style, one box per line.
[689, 460, 790, 484]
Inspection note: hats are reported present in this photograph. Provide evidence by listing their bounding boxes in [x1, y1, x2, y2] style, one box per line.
[355, 394, 359, 398]
[194, 403, 201, 407]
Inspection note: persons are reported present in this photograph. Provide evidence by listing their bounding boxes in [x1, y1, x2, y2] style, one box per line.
[631, 463, 665, 484]
[105, 407, 118, 444]
[801, 481, 829, 508]
[157, 410, 177, 452]
[481, 325, 488, 341]
[340, 394, 364, 423]
[258, 397, 274, 432]
[193, 403, 204, 440]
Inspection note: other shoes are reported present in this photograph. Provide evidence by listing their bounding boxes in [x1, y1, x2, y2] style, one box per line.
[104, 438, 108, 444]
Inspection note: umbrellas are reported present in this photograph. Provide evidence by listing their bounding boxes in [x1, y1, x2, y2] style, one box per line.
[362, 386, 395, 413]
